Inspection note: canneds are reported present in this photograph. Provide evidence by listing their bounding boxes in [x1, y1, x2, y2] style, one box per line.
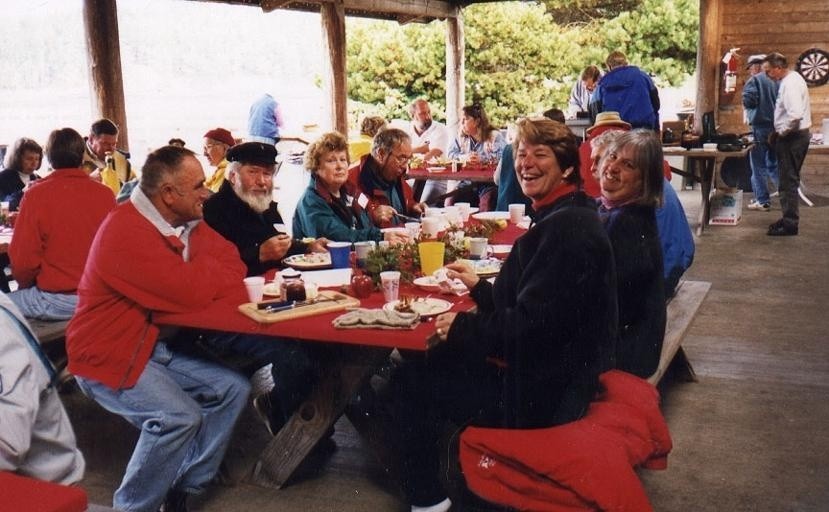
[280, 274, 305, 305]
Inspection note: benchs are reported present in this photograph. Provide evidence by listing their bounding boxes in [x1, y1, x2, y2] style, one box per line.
[626, 274, 711, 398]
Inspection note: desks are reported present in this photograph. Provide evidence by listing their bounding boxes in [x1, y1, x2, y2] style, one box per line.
[0, 469, 88, 512]
[769, 140, 829, 206]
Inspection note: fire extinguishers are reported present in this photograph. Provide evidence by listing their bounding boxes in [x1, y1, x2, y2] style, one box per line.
[723, 48, 742, 97]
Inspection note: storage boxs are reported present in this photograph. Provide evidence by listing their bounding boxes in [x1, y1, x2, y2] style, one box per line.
[709, 185, 745, 227]
[662, 114, 690, 146]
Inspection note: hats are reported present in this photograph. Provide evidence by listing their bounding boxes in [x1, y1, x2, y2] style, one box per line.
[585, 109, 632, 130]
[202, 127, 237, 147]
[226, 142, 277, 166]
[743, 52, 764, 69]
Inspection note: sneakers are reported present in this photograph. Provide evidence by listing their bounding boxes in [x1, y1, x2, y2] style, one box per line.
[748, 196, 799, 238]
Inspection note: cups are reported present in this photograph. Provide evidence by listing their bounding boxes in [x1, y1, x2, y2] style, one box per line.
[0, 202, 10, 218]
[243, 276, 265, 304]
[405, 201, 527, 260]
[327, 241, 466, 302]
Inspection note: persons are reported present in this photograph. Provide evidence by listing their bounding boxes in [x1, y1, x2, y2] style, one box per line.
[580, 125, 664, 378]
[289, 132, 410, 376]
[6, 127, 116, 320]
[589, 51, 660, 129]
[65, 146, 250, 511]
[251, 88, 285, 140]
[200, 141, 333, 405]
[444, 104, 507, 211]
[400, 99, 449, 208]
[742, 54, 780, 211]
[0, 285, 87, 487]
[762, 53, 812, 236]
[343, 116, 621, 511]
[569, 65, 607, 117]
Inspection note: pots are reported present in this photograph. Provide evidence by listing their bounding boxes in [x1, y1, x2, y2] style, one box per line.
[713, 132, 756, 153]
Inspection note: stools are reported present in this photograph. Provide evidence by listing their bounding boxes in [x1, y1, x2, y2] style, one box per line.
[30, 314, 78, 399]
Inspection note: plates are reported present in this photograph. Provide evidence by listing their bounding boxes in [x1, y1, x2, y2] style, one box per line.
[262, 281, 319, 297]
[380, 228, 404, 233]
[386, 297, 452, 316]
[414, 156, 496, 172]
[455, 260, 502, 276]
[283, 252, 333, 267]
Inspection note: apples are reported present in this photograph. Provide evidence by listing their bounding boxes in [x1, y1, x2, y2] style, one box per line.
[352, 275, 372, 297]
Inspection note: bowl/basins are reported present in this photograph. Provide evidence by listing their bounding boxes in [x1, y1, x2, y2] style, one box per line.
[704, 143, 717, 151]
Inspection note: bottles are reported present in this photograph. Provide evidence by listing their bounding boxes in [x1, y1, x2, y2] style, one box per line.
[100, 151, 120, 195]
[278, 273, 305, 303]
[682, 121, 693, 149]
